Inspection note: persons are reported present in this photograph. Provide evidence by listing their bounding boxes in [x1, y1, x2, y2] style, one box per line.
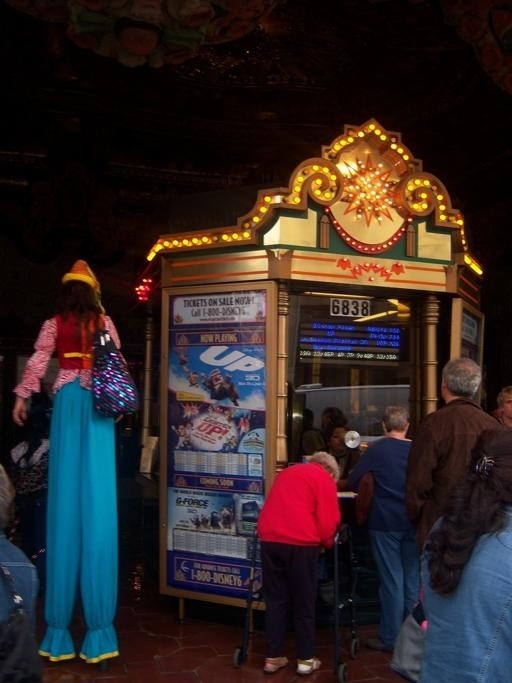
[171, 326, 265, 532]
[0, 463, 43, 683]
[256, 356, 512, 683]
[12, 260, 123, 674]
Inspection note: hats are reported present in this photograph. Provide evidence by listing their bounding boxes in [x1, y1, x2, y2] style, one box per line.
[61, 259, 101, 290]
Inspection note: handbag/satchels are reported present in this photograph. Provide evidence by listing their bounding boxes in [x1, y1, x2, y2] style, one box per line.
[91, 326, 141, 417]
[391, 605, 426, 682]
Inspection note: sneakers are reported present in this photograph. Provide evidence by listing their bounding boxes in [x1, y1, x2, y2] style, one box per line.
[263, 656, 290, 674]
[365, 636, 392, 649]
[296, 654, 322, 673]
